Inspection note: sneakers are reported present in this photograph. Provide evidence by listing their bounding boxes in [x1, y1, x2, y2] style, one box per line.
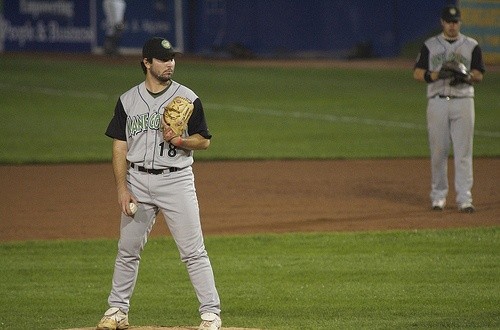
[96, 308, 129, 330]
[197, 313, 222, 330]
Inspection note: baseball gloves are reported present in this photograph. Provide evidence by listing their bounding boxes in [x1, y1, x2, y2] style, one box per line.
[160, 96, 194, 143]
[440, 57, 471, 80]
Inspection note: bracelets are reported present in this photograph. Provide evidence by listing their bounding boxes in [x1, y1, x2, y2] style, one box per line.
[424, 71, 434, 83]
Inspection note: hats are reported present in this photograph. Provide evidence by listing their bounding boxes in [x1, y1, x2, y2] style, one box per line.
[441, 6, 462, 22]
[142, 36, 184, 62]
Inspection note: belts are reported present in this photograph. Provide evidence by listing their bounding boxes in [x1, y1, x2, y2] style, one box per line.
[432, 95, 456, 98]
[131, 164, 179, 174]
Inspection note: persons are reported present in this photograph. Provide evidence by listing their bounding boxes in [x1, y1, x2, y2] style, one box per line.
[98, 37, 223, 330]
[412, 5, 486, 213]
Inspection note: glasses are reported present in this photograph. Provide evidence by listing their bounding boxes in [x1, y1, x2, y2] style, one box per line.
[446, 19, 459, 24]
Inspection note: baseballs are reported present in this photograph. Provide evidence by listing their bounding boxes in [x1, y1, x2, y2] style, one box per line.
[130, 203, 138, 215]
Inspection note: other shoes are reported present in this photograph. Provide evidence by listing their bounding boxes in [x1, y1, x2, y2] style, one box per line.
[432, 197, 447, 210]
[457, 203, 475, 213]
